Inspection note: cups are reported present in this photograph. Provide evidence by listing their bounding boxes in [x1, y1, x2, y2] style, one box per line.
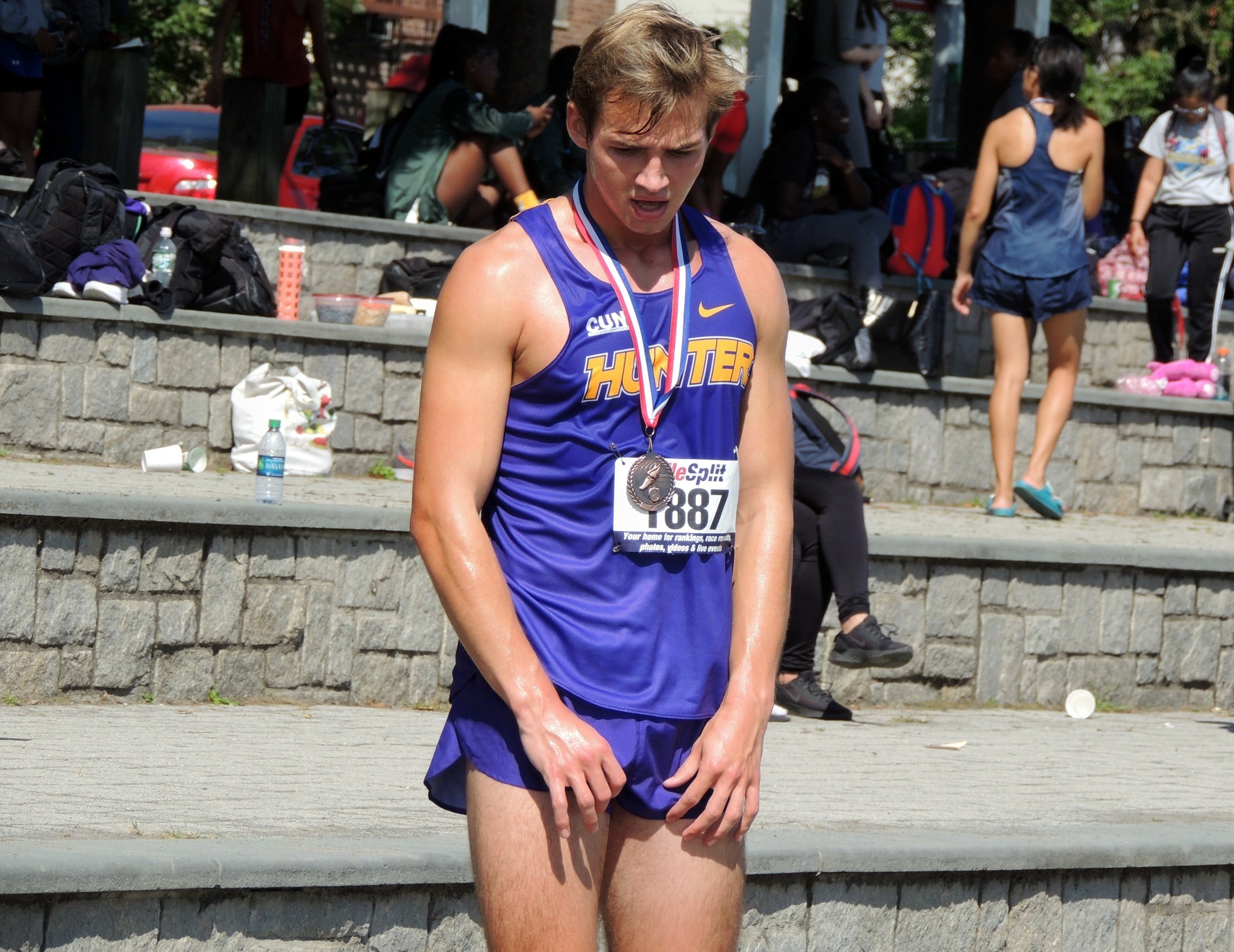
[1065, 689, 1096, 719]
[182, 447, 207, 473]
[141, 444, 183, 474]
[1108, 278, 1121, 299]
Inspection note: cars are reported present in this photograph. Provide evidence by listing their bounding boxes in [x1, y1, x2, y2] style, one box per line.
[137, 103, 386, 219]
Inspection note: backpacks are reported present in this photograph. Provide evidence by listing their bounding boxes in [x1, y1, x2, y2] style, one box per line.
[888, 169, 955, 293]
[18, 158, 134, 280]
[0, 212, 49, 298]
[134, 202, 278, 317]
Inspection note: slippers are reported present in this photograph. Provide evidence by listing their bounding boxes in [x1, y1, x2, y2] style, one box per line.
[1014, 478, 1065, 520]
[986, 493, 1018, 517]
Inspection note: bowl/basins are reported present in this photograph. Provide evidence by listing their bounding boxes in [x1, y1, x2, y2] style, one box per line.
[312, 294, 395, 328]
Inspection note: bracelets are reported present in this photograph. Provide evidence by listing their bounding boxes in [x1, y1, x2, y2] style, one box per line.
[1129, 219, 1143, 225]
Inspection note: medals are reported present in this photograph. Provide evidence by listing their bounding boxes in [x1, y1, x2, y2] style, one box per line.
[626, 450, 674, 511]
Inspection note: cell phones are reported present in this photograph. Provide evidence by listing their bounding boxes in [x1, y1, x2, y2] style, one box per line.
[540, 95, 557, 108]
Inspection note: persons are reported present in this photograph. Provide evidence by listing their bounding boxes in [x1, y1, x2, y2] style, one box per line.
[408, 2, 796, 952]
[201, 0, 339, 177]
[773, 462, 914, 721]
[0, 0, 148, 180]
[527, 44, 588, 197]
[1127, 44, 1234, 363]
[951, 36, 1105, 521]
[386, 22, 554, 228]
[809, 0, 892, 174]
[985, 24, 1038, 125]
[685, 23, 748, 222]
[745, 74, 894, 315]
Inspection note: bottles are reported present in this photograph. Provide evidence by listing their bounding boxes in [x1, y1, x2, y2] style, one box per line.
[1213, 347, 1231, 400]
[254, 419, 286, 504]
[275, 245, 306, 321]
[152, 227, 177, 289]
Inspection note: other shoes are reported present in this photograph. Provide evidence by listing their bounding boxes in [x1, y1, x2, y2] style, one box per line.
[52, 282, 83, 299]
[81, 280, 130, 305]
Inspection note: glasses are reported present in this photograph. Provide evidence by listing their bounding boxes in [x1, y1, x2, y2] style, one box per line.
[1173, 103, 1208, 116]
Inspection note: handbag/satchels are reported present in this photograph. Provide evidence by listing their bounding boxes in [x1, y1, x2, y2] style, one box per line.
[376, 258, 457, 300]
[1096, 234, 1150, 299]
[902, 288, 947, 377]
[232, 363, 336, 476]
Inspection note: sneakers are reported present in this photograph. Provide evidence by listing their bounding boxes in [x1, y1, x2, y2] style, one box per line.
[828, 615, 914, 669]
[775, 669, 852, 721]
